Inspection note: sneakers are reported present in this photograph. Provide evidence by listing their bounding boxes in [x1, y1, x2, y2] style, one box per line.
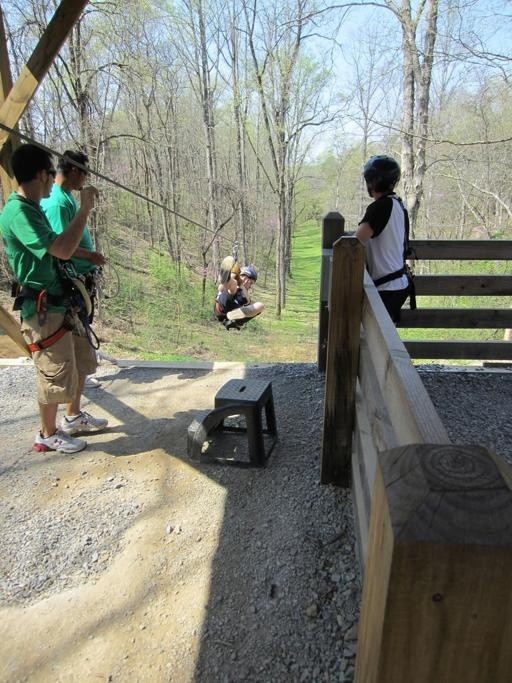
[215, 254, 236, 284]
[61, 411, 109, 437]
[84, 377, 100, 390]
[227, 306, 252, 321]
[32, 426, 87, 455]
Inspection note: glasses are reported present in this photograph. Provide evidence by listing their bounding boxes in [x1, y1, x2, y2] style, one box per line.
[41, 165, 58, 179]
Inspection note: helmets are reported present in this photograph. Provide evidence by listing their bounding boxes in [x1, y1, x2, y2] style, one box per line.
[362, 154, 403, 187]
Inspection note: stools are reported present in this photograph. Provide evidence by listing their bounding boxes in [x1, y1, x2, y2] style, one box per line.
[185, 379, 278, 468]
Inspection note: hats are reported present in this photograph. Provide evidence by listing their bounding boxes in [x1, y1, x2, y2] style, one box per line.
[240, 266, 257, 280]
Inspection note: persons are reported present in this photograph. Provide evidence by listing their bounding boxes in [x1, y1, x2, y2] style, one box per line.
[40, 145, 106, 388]
[352, 148, 412, 320]
[212, 254, 267, 329]
[0, 143, 110, 454]
